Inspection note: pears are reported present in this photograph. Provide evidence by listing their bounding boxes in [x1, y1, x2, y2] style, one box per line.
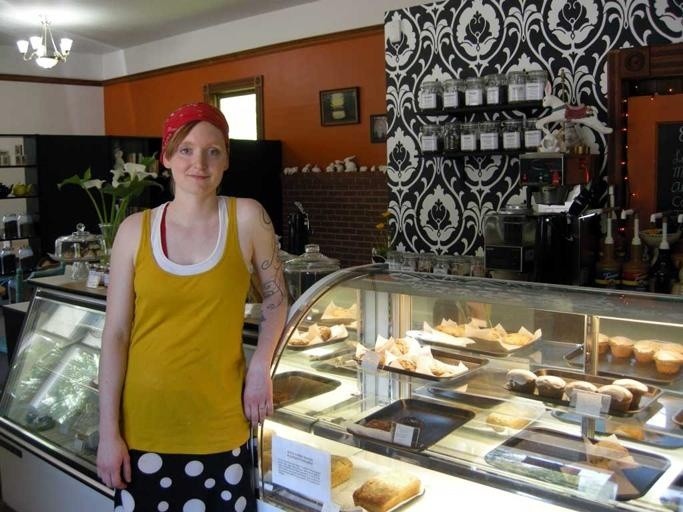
[26, 183, 37, 195]
[13, 179, 26, 196]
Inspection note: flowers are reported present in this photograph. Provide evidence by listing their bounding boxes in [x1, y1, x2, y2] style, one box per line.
[373, 212, 388, 256]
[57, 152, 171, 266]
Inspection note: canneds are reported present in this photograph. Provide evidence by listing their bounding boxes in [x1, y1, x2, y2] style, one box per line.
[87, 263, 110, 288]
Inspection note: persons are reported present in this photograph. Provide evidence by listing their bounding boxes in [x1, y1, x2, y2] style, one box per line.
[96, 101, 288, 512]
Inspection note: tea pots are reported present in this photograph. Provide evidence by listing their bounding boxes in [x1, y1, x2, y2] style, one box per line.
[0, 181, 15, 199]
[12, 181, 35, 200]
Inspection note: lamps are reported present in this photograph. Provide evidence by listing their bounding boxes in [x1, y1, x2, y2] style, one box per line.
[16, 14, 74, 68]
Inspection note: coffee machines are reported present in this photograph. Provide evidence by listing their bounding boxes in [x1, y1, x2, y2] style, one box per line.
[481, 149, 602, 284]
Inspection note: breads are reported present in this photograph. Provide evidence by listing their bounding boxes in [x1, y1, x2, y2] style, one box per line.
[353, 472, 421, 512]
[331, 455, 353, 488]
[592, 440, 629, 469]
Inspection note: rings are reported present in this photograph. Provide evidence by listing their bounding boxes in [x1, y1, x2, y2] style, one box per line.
[260, 405, 266, 410]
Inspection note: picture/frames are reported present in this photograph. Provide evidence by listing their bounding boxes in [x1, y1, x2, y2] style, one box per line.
[320, 86, 359, 126]
[370, 114, 387, 143]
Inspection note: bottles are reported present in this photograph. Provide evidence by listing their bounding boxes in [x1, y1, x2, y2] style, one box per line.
[385, 249, 486, 280]
[16, 213, 34, 239]
[15, 144, 29, 166]
[0, 151, 11, 166]
[8, 278, 16, 304]
[415, 68, 550, 154]
[588, 206, 623, 291]
[1, 212, 18, 239]
[282, 242, 340, 309]
[0, 247, 18, 275]
[88, 262, 111, 288]
[643, 210, 679, 296]
[670, 214, 683, 295]
[18, 246, 35, 271]
[616, 208, 651, 293]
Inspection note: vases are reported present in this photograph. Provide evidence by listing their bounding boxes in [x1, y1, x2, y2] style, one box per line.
[372, 248, 387, 264]
[97, 223, 116, 273]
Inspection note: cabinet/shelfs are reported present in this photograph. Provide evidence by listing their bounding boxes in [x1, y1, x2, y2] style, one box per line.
[0, 272, 260, 512]
[0, 133, 40, 278]
[414, 100, 542, 158]
[251, 265, 681, 510]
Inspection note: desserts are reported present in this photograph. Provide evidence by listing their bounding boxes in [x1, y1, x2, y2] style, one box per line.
[615, 424, 645, 441]
[508, 367, 649, 411]
[364, 420, 390, 431]
[404, 416, 425, 430]
[369, 339, 453, 378]
[436, 321, 534, 350]
[598, 334, 682, 374]
